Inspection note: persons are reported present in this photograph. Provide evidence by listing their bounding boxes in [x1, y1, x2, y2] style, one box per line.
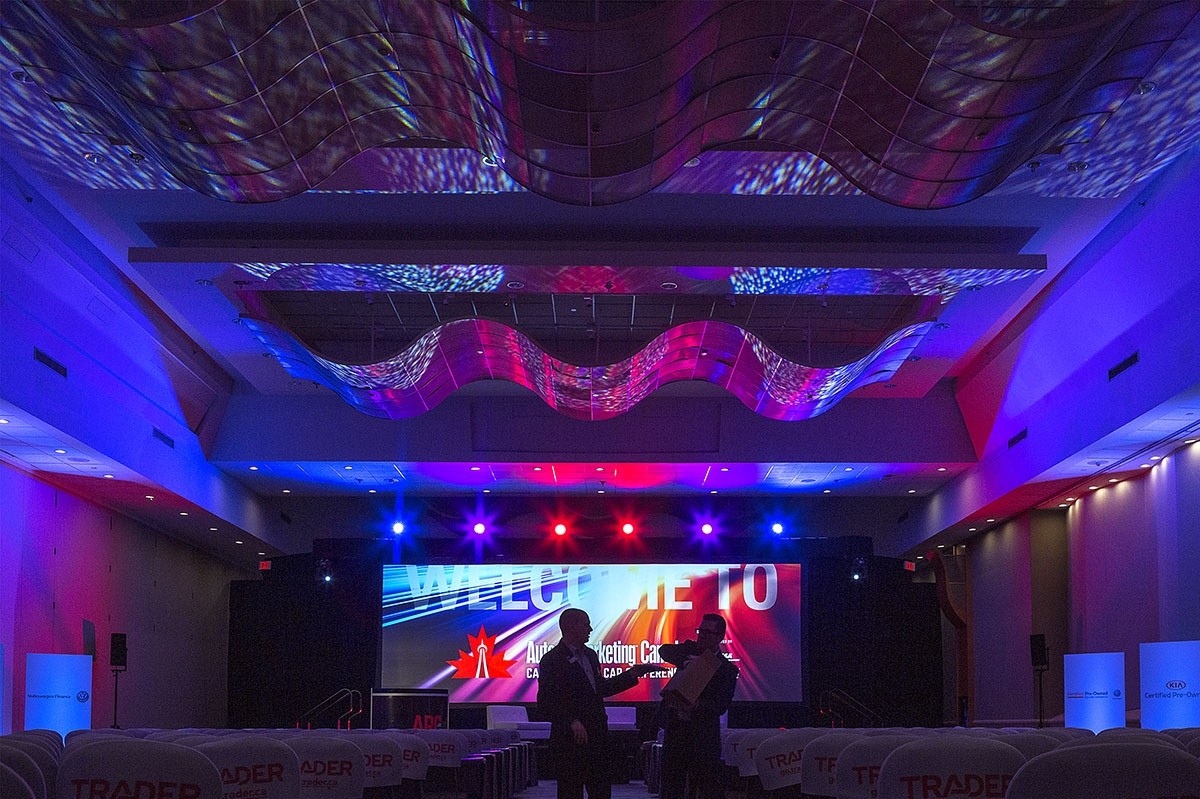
[537, 608, 653, 799]
[658, 613, 740, 799]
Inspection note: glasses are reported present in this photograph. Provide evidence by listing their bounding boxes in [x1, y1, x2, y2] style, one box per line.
[696, 629, 720, 636]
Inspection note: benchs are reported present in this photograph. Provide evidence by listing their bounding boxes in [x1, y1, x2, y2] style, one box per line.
[487, 706, 551, 738]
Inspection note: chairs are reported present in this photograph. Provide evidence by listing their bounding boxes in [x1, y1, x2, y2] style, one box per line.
[639, 726, 1200, 799]
[605, 707, 636, 731]
[0, 728, 535, 799]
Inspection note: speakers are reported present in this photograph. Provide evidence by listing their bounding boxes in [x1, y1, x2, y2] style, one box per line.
[109, 632, 127, 667]
[1030, 633, 1049, 671]
